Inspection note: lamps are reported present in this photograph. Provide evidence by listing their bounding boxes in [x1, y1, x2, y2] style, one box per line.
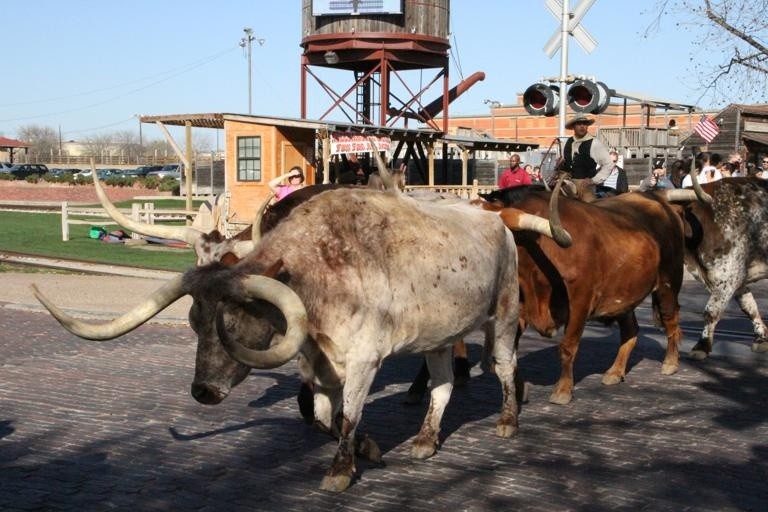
[323, 50, 340, 64]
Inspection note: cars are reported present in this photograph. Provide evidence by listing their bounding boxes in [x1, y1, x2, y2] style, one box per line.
[0, 162, 194, 181]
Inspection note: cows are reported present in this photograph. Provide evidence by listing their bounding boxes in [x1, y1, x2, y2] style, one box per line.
[368, 138, 686, 406]
[26, 186, 520, 494]
[89, 157, 468, 428]
[651, 156, 768, 361]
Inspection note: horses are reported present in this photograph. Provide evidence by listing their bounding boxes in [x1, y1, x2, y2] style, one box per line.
[368, 162, 407, 191]
[476, 184, 622, 210]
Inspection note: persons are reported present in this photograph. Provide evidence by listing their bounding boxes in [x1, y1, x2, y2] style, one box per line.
[268, 166, 306, 202]
[497, 112, 768, 202]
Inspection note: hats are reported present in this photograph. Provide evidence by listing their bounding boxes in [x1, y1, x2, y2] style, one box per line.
[565, 111, 595, 129]
